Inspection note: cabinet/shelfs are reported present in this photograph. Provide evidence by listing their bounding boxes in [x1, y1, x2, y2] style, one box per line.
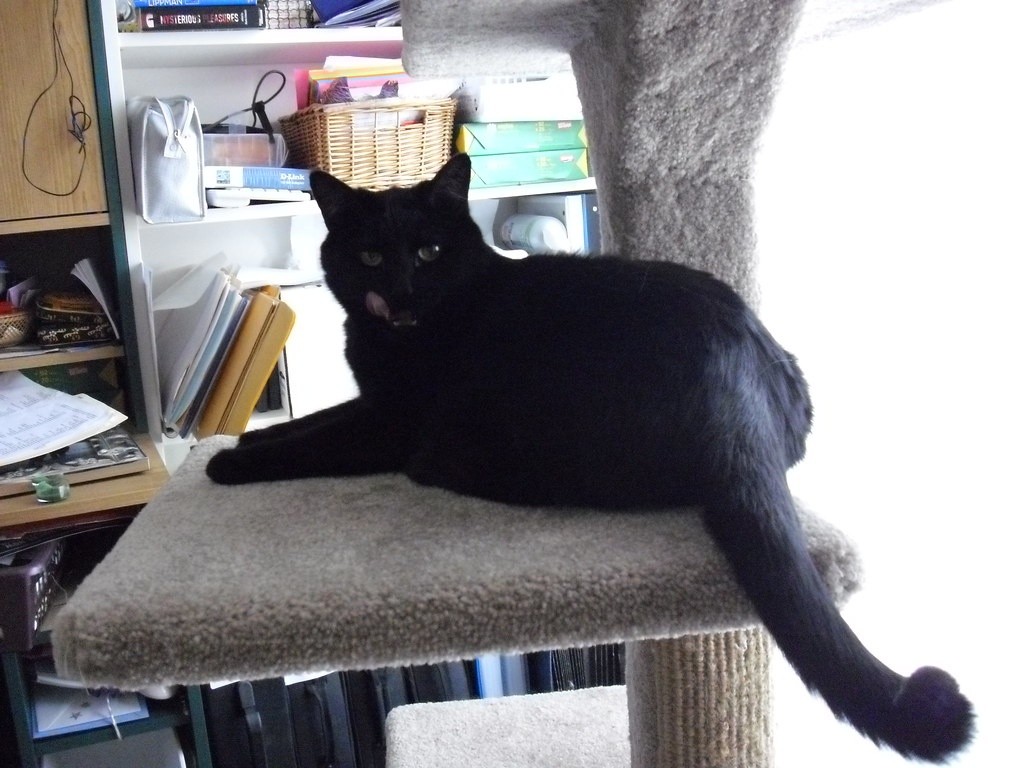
[0, 0, 865, 768]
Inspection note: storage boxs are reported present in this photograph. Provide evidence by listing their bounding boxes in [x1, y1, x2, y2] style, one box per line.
[452, 119, 588, 156]
[271, 282, 361, 417]
[468, 147, 588, 190]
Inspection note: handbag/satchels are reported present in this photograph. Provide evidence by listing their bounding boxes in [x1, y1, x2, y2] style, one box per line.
[127, 94, 208, 224]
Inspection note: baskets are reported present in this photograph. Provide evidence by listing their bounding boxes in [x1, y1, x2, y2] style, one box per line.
[0, 311, 32, 348]
[278, 98, 460, 192]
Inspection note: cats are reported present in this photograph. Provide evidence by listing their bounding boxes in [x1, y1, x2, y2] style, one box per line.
[204, 153, 976, 766]
